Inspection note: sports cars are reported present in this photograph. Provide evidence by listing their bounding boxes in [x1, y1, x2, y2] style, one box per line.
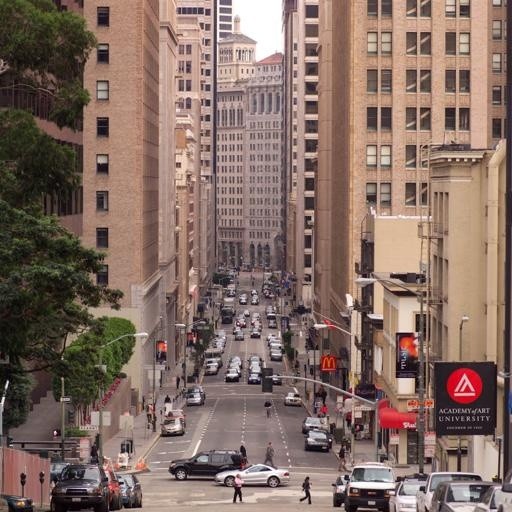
[213, 462, 293, 488]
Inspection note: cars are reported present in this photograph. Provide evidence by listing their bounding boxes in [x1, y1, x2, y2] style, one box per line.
[46, 463, 144, 512]
[158, 383, 206, 435]
[330, 458, 512, 512]
[0, 492, 35, 512]
[284, 390, 334, 453]
[202, 265, 286, 386]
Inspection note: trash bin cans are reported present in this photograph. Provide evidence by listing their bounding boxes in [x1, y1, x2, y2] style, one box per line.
[333, 428, 343, 444]
[7, 498, 35, 512]
[296, 370, 300, 382]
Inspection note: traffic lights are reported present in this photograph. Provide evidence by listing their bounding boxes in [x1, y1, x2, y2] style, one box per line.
[52, 429, 58, 437]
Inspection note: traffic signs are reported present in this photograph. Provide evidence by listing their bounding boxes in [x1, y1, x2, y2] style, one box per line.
[58, 396, 71, 403]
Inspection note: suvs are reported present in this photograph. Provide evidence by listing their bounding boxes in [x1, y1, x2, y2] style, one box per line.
[165, 449, 244, 482]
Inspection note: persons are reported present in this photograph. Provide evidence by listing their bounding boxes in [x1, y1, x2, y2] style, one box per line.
[176, 376, 180, 389]
[233, 474, 245, 503]
[95, 433, 99, 450]
[264, 442, 277, 470]
[300, 476, 312, 504]
[264, 400, 272, 418]
[231, 440, 249, 470]
[312, 384, 328, 417]
[346, 410, 352, 430]
[90, 441, 100, 466]
[310, 365, 314, 376]
[147, 403, 153, 425]
[338, 444, 354, 472]
[164, 395, 171, 412]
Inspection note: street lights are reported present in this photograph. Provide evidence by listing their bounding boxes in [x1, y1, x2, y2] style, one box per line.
[353, 275, 429, 479]
[266, 310, 296, 385]
[226, 255, 244, 270]
[0, 378, 9, 437]
[311, 322, 359, 377]
[183, 318, 206, 389]
[151, 322, 187, 404]
[286, 319, 317, 410]
[97, 331, 149, 464]
[454, 314, 470, 473]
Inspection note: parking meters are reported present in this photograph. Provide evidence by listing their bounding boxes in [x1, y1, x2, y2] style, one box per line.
[20, 472, 27, 500]
[38, 470, 45, 508]
